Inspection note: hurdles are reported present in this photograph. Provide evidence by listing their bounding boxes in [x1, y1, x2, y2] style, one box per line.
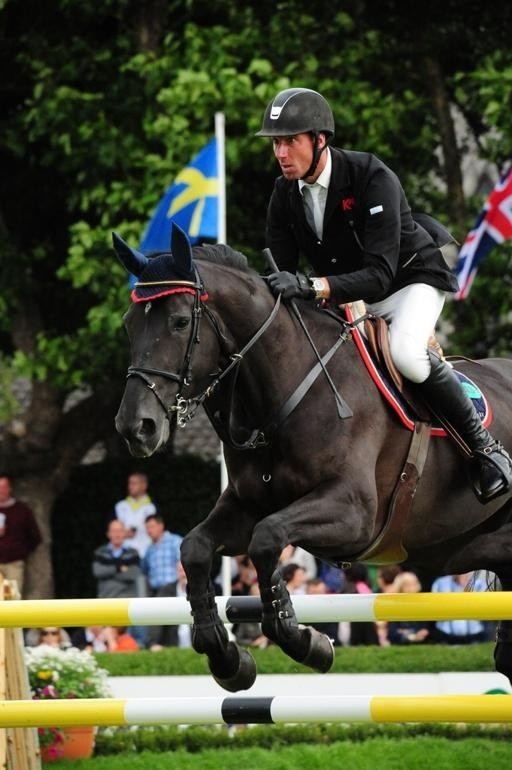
[0, 579, 512, 770]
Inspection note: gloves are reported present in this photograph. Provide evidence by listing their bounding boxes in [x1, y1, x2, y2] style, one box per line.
[266, 270, 316, 307]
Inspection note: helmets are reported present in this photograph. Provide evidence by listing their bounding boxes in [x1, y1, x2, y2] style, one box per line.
[255, 87, 334, 139]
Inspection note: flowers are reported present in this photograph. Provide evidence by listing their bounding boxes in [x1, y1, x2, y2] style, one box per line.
[24, 644, 109, 761]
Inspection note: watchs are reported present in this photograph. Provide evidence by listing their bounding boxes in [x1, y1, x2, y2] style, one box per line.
[310, 277, 325, 300]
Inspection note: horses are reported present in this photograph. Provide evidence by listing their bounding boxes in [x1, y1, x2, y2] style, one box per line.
[112, 221, 512, 693]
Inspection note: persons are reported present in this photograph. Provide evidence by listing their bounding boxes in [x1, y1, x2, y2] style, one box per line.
[254, 87, 511, 504]
[0, 472, 491, 652]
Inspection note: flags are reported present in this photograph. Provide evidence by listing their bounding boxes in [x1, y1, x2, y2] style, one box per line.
[127, 135, 219, 285]
[445, 165, 511, 300]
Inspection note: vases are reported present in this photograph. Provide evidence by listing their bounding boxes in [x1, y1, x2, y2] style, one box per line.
[48, 724, 97, 761]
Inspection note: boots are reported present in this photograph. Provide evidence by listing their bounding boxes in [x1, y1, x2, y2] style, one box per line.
[402, 347, 511, 506]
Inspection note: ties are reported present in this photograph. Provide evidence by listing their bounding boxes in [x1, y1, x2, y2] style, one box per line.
[304, 185, 325, 241]
